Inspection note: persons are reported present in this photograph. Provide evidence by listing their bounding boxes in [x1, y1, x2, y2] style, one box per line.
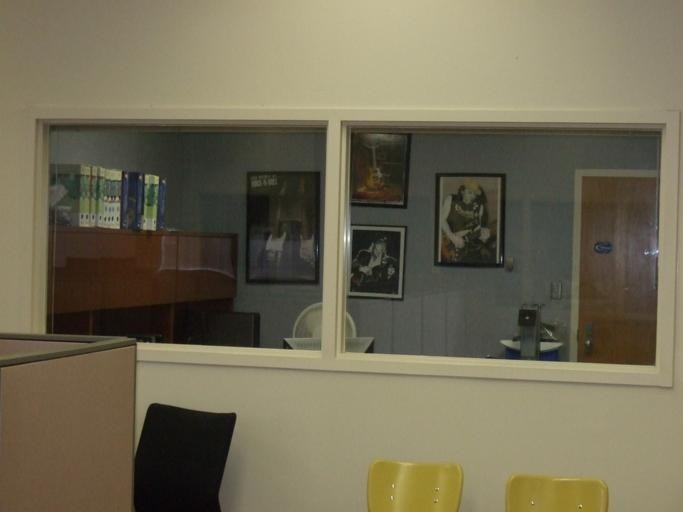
[352, 236, 398, 294]
[441, 184, 496, 264]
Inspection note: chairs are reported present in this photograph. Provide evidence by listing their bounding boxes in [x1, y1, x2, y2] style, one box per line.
[366, 460, 607, 512]
[134, 397, 238, 511]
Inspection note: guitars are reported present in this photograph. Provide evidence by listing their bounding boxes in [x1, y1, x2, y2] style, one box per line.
[262, 180, 285, 267]
[367, 147, 386, 189]
[299, 179, 315, 260]
[442, 220, 496, 264]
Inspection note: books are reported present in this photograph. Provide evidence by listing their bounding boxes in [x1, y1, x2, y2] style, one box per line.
[53, 165, 168, 231]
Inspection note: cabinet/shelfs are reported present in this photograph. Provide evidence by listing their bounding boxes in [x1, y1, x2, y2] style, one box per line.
[49, 227, 238, 343]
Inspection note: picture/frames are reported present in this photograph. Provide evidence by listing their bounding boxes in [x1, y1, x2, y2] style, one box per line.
[347, 128, 411, 300]
[433, 172, 510, 268]
[245, 170, 319, 287]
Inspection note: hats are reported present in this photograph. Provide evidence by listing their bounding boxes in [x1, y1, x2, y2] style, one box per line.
[464, 179, 481, 196]
